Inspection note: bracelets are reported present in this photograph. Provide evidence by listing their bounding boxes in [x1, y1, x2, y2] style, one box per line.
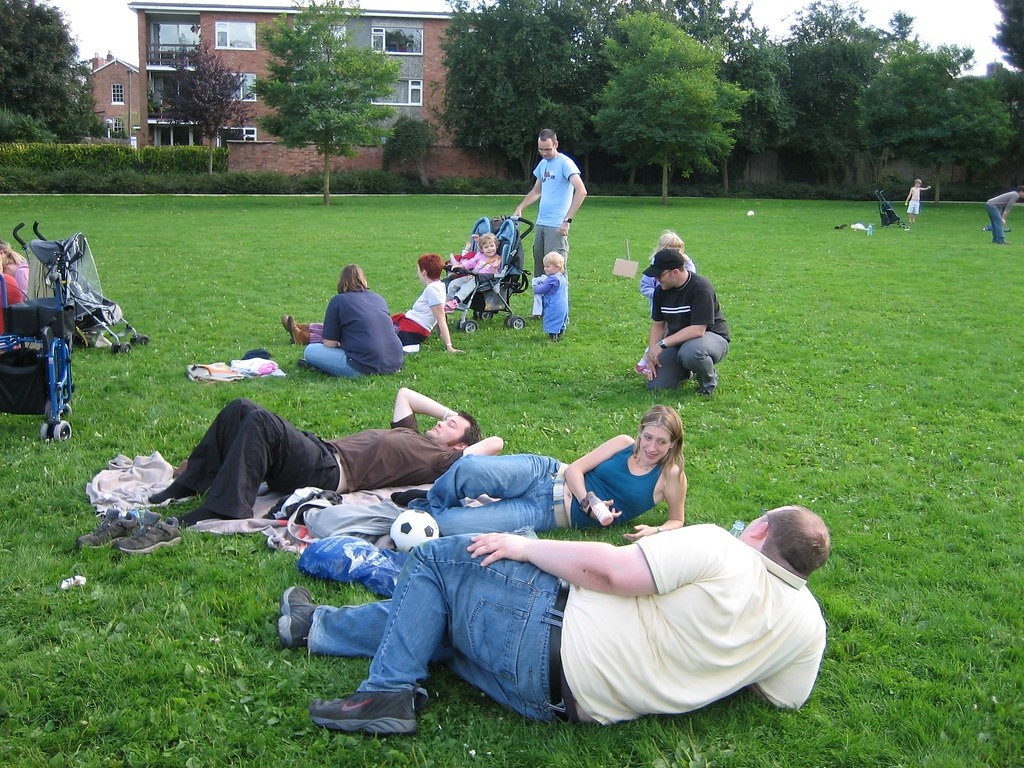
[657, 526, 664, 532]
[441, 407, 449, 420]
[587, 507, 592, 516]
[446, 343, 452, 346]
[579, 496, 589, 512]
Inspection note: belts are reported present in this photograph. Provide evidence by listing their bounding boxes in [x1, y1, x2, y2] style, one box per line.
[549, 581, 571, 722]
[552, 464, 568, 529]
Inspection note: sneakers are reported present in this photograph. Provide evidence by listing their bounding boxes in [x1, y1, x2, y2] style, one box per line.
[308, 691, 417, 734]
[117, 510, 182, 554]
[278, 586, 316, 648]
[76, 508, 140, 548]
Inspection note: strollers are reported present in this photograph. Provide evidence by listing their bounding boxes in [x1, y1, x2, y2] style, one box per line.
[0, 221, 149, 444]
[874, 190, 909, 229]
[442, 215, 534, 335]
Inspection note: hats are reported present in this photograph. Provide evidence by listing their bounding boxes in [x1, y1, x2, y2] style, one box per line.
[642, 249, 685, 277]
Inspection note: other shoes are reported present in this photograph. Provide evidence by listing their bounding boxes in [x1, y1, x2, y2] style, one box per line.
[694, 386, 714, 396]
[447, 300, 458, 309]
[444, 306, 454, 314]
[530, 315, 540, 320]
[297, 358, 325, 373]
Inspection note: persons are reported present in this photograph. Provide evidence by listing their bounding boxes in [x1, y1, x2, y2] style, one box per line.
[281, 253, 466, 353]
[640, 229, 696, 314]
[986, 186, 1024, 244]
[149, 387, 504, 529]
[444, 233, 501, 314]
[450, 234, 480, 265]
[513, 129, 587, 319]
[0, 238, 29, 336]
[297, 264, 403, 377]
[905, 179, 931, 224]
[533, 251, 569, 342]
[278, 503, 830, 733]
[391, 405, 688, 543]
[642, 249, 730, 395]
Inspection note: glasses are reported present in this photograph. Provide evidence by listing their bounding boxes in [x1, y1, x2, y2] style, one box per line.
[537, 144, 554, 152]
[655, 270, 671, 278]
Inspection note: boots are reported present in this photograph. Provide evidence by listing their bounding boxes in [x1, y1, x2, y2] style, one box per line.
[287, 315, 310, 345]
[282, 315, 309, 333]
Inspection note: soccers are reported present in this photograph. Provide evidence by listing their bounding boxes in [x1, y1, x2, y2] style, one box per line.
[389, 508, 439, 553]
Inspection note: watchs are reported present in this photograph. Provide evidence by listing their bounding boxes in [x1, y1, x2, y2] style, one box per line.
[564, 217, 572, 223]
[658, 339, 667, 350]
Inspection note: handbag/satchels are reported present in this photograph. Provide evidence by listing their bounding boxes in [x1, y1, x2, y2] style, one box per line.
[287, 498, 401, 544]
[186, 360, 246, 381]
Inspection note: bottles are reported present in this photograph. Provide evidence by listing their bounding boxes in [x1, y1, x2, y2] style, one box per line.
[728, 519, 746, 539]
[586, 491, 613, 526]
[868, 224, 874, 235]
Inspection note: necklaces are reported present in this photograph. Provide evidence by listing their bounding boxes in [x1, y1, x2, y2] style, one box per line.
[634, 456, 654, 470]
